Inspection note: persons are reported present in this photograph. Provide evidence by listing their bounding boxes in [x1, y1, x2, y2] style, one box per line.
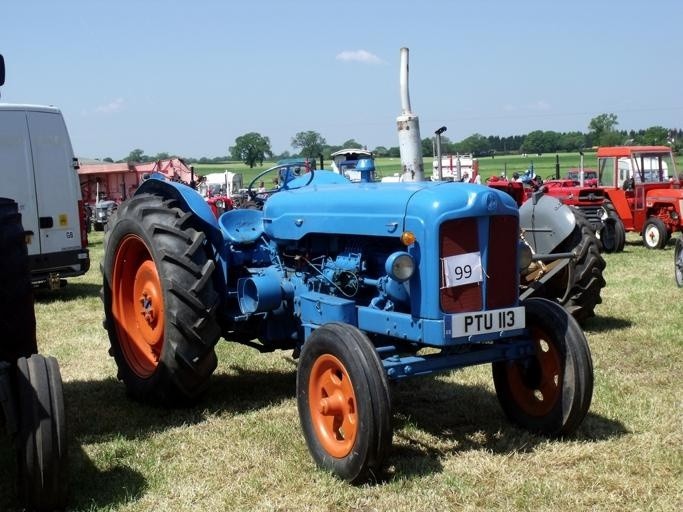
[271, 178, 281, 190]
[510, 172, 522, 183]
[257, 181, 269, 198]
[522, 170, 532, 183]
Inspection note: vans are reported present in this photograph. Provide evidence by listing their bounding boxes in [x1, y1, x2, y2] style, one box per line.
[0, 102, 93, 290]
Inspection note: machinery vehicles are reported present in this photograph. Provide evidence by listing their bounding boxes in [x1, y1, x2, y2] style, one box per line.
[592, 142, 683, 251]
[488, 149, 608, 249]
